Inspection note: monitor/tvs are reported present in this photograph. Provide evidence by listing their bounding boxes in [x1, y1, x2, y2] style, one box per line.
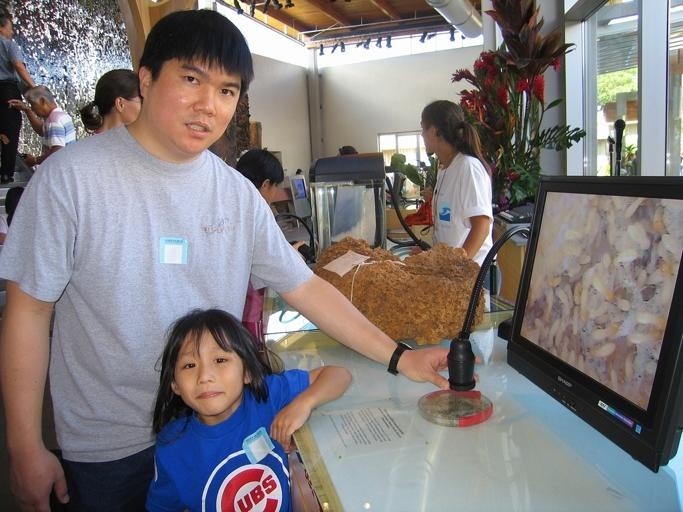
[506, 174, 683, 474]
[292, 178, 308, 200]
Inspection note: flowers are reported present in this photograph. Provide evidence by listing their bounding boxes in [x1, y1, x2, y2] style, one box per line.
[390, 0, 586, 227]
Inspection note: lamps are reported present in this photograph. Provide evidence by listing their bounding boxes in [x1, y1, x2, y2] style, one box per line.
[232, 0, 295, 18]
[310, 19, 467, 55]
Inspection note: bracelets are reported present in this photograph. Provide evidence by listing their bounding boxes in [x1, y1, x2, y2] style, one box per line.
[388, 340, 414, 374]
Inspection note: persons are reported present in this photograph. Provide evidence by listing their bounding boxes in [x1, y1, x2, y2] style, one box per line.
[419, 100, 503, 292]
[336, 145, 358, 156]
[294, 168, 307, 178]
[233, 147, 305, 342]
[1, 1, 141, 248]
[1, 11, 482, 512]
[146, 309, 353, 512]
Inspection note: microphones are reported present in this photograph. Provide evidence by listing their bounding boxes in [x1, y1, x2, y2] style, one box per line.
[614, 119, 625, 160]
